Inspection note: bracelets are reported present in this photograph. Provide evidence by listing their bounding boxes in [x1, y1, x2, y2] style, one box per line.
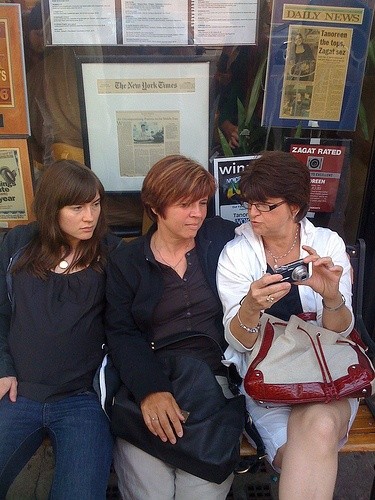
[322, 292, 345, 312]
[237, 310, 260, 333]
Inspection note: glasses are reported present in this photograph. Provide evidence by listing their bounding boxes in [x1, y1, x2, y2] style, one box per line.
[240, 199, 288, 212]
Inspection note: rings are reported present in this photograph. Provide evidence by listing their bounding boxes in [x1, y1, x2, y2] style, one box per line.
[150, 416, 158, 420]
[266, 295, 275, 302]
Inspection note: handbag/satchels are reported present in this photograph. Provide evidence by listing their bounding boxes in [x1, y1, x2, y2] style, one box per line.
[93, 332, 267, 485]
[243, 311, 375, 410]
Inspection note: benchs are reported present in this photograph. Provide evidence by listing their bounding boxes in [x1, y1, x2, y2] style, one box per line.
[111, 230, 374, 458]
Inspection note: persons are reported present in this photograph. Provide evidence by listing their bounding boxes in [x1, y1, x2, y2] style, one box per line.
[290, 33, 316, 80]
[105, 152, 243, 500]
[0, 159, 126, 500]
[215, 150, 360, 500]
[25, 0, 267, 151]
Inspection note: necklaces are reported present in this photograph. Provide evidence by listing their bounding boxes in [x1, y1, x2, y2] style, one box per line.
[59, 260, 68, 269]
[154, 233, 190, 268]
[265, 224, 298, 268]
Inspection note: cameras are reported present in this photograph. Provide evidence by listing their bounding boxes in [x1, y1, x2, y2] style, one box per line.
[274, 259, 309, 285]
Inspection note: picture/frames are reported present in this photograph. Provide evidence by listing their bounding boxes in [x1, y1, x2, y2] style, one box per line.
[0, 4, 37, 231]
[74, 54, 215, 198]
[212, 154, 261, 225]
[282, 137, 352, 218]
